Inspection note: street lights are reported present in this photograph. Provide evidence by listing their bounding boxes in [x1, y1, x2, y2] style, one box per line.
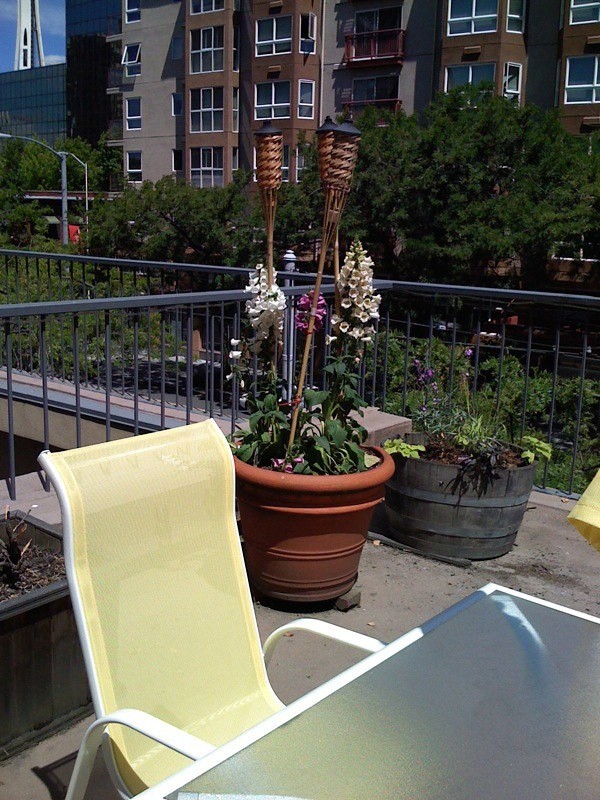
[57, 151, 90, 300]
[0, 132, 70, 273]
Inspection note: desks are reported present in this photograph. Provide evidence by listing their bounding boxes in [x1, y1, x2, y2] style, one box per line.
[140, 582, 600, 800]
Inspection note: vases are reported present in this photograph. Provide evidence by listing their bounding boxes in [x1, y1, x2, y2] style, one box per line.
[392, 443, 531, 563]
[227, 442, 395, 607]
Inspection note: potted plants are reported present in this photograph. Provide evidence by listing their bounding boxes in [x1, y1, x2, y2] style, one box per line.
[0, 504, 95, 762]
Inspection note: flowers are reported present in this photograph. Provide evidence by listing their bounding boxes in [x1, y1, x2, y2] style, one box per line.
[230, 242, 397, 473]
[389, 310, 541, 470]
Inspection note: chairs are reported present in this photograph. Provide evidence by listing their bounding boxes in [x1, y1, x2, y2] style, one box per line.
[38, 419, 385, 800]
